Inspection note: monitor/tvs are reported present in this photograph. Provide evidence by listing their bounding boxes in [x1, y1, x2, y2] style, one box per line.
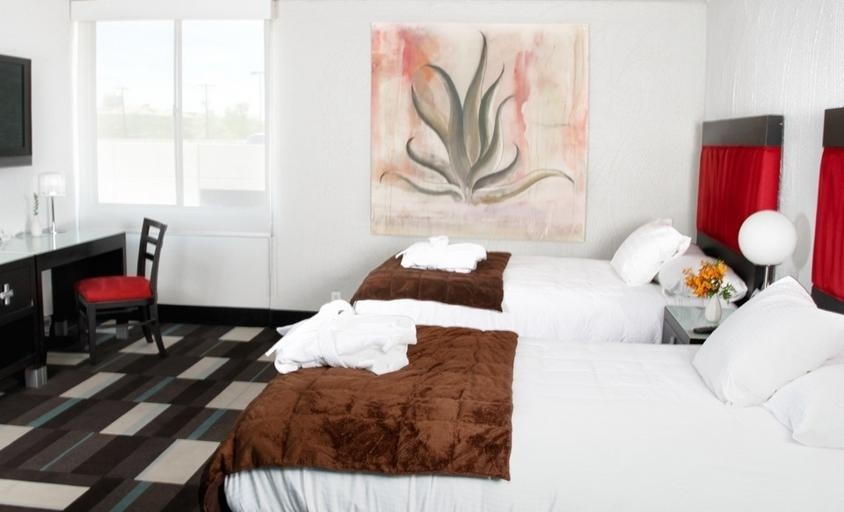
[0, 55, 31, 167]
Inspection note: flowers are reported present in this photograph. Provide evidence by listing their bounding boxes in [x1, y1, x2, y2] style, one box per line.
[681, 258, 737, 304]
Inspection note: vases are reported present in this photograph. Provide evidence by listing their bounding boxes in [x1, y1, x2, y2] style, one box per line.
[704, 293, 721, 322]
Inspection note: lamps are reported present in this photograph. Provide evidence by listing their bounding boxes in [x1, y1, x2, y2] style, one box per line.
[738, 210, 797, 295]
[38, 171, 68, 235]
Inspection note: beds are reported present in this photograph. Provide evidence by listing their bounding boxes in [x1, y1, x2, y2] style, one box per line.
[205, 107, 844, 512]
[350, 115, 783, 342]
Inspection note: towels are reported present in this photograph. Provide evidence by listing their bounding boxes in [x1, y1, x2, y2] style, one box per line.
[270, 300, 419, 374]
[400, 228, 486, 274]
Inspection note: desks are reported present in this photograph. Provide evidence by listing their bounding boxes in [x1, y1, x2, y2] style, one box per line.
[0, 222, 128, 396]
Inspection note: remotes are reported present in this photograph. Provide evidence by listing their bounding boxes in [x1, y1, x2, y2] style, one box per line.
[694, 327, 717, 333]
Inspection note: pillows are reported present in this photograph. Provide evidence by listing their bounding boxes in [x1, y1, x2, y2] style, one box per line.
[610, 216, 692, 287]
[691, 275, 844, 409]
[762, 357, 844, 445]
[653, 244, 748, 305]
[814, 307, 844, 364]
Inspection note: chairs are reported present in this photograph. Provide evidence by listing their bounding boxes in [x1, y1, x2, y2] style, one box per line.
[74, 217, 167, 366]
